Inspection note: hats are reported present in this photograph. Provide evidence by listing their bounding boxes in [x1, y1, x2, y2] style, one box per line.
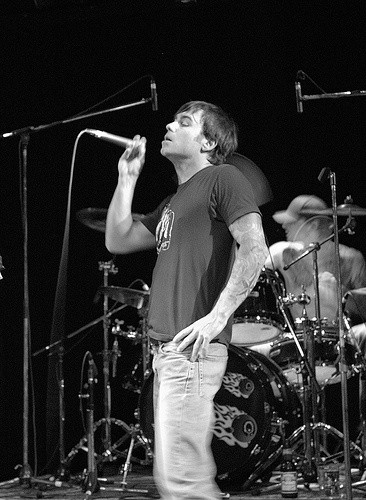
[273, 196, 327, 226]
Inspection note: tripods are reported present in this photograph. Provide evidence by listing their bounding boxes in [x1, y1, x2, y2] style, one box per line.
[0, 94, 154, 499]
[240, 217, 366, 498]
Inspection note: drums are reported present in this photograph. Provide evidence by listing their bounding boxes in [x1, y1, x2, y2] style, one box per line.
[267, 320, 359, 387]
[105, 327, 146, 397]
[227, 267, 287, 348]
[137, 344, 304, 491]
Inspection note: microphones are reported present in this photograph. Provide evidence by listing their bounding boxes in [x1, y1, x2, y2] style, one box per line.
[85, 129, 140, 159]
[335, 292, 349, 325]
[151, 80, 158, 110]
[295, 78, 303, 113]
[88, 352, 98, 376]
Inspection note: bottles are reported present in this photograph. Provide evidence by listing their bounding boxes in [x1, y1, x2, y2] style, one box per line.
[281, 440, 297, 497]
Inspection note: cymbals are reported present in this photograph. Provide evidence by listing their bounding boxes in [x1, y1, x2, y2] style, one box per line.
[73, 206, 147, 234]
[93, 284, 150, 311]
[349, 287, 366, 296]
[294, 204, 366, 216]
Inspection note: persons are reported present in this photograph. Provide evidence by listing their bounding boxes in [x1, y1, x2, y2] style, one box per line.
[105, 100, 270, 500]
[263, 195, 366, 323]
